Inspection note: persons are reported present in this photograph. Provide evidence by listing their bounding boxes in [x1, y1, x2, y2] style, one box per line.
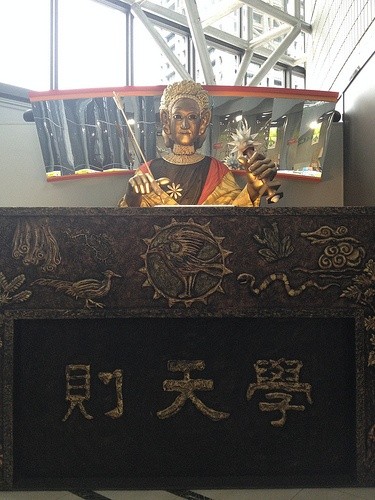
[117, 81, 275, 207]
